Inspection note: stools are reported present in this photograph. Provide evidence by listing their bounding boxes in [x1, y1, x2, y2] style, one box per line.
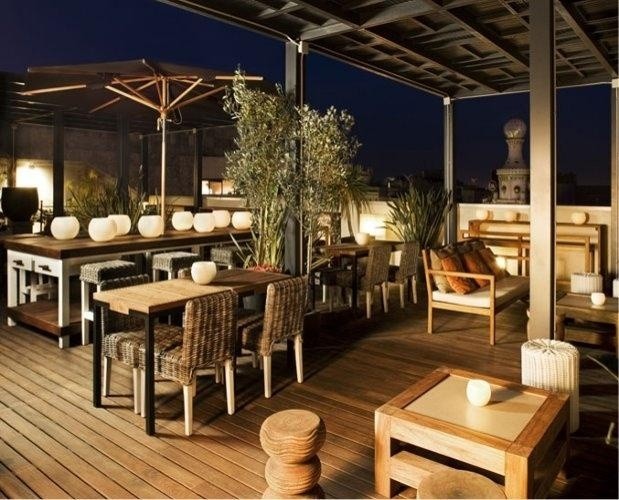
[520, 337, 581, 435]
[417, 469, 507, 499]
[570, 272, 603, 325]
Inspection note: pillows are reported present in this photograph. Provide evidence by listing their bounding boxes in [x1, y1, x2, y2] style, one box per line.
[428, 241, 509, 296]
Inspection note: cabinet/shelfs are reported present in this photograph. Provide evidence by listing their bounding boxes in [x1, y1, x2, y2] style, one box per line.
[468, 219, 602, 277]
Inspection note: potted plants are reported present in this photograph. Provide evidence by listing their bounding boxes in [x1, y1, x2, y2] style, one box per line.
[222, 63, 362, 312]
[377, 173, 454, 304]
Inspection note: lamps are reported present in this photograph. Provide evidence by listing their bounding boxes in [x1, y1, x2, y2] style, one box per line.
[466, 377, 492, 406]
[475, 207, 489, 222]
[570, 209, 586, 226]
[503, 209, 516, 222]
[49, 209, 253, 244]
[591, 292, 607, 306]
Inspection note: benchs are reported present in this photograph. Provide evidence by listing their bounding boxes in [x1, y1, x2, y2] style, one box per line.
[421, 236, 530, 346]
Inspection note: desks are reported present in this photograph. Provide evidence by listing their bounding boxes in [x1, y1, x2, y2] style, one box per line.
[0, 227, 260, 349]
[374, 365, 570, 500]
[555, 291, 619, 358]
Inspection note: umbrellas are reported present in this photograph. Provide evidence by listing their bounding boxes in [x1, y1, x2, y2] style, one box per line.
[22, 58, 277, 237]
